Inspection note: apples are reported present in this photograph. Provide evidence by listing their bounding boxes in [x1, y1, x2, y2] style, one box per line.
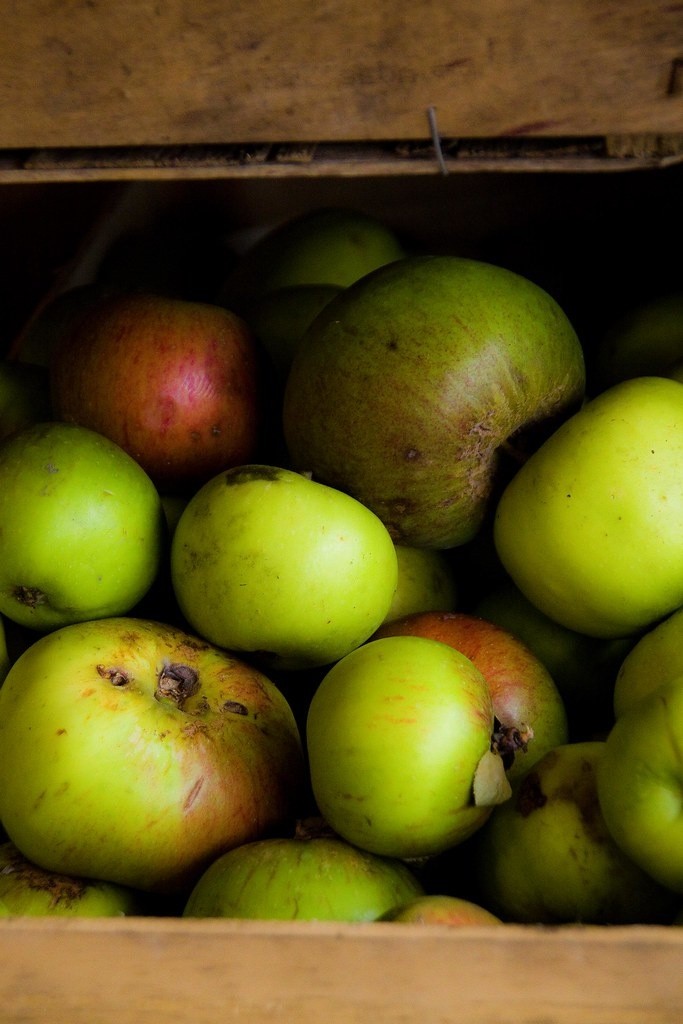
[0, 215, 683, 926]
